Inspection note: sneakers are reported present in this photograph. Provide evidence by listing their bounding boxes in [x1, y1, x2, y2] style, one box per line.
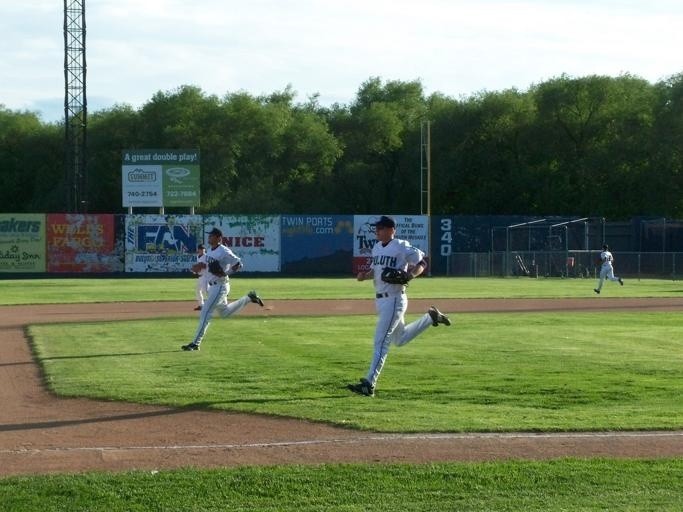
[348, 379, 374, 398]
[247, 290, 263, 307]
[427, 306, 450, 326]
[181, 344, 200, 351]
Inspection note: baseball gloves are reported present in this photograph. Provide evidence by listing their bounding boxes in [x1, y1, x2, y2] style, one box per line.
[208, 261, 226, 277]
[380, 267, 410, 285]
[191, 262, 204, 274]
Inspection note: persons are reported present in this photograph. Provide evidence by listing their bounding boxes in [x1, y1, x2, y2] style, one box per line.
[594, 244, 623, 294]
[155, 216, 195, 254]
[194, 244, 209, 310]
[180, 227, 264, 351]
[348, 217, 451, 398]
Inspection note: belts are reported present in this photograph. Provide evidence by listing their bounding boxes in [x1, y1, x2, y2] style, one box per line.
[376, 291, 404, 298]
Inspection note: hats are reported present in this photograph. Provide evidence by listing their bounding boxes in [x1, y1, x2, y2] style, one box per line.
[205, 228, 221, 236]
[370, 216, 395, 227]
